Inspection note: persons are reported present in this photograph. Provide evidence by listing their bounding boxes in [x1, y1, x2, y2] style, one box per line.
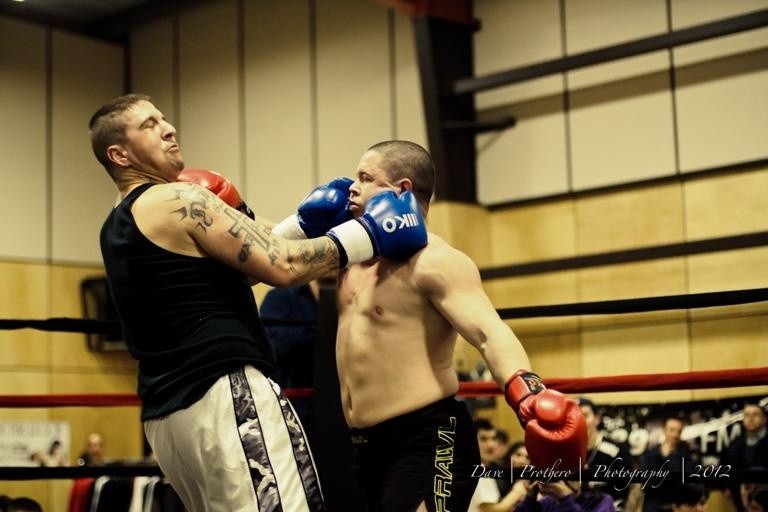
[175, 139, 589, 511]
[87, 93, 428, 511]
[469, 394, 768, 511]
[0, 432, 187, 512]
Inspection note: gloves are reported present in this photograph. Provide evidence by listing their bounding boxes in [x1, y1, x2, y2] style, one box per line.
[271, 178, 355, 242]
[175, 169, 256, 221]
[325, 190, 427, 271]
[504, 368, 590, 473]
